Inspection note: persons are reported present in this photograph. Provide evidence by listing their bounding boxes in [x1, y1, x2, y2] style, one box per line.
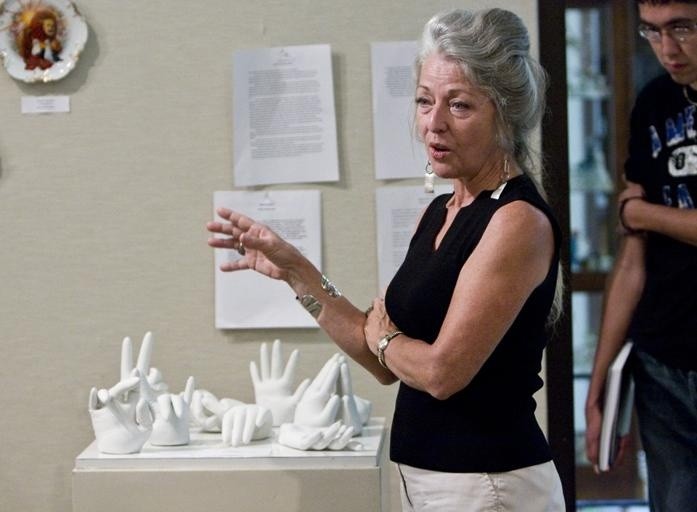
[583, 0, 696, 512]
[203, 7, 572, 511]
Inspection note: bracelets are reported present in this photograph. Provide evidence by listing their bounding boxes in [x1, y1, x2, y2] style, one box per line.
[297, 275, 342, 319]
[617, 195, 640, 234]
[376, 331, 404, 370]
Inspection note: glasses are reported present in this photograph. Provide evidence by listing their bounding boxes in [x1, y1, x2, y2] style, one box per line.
[638, 24, 697, 43]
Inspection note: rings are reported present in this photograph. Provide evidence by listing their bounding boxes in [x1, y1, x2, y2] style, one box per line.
[237, 241, 245, 255]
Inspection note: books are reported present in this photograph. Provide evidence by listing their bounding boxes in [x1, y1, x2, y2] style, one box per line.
[597, 341, 634, 473]
